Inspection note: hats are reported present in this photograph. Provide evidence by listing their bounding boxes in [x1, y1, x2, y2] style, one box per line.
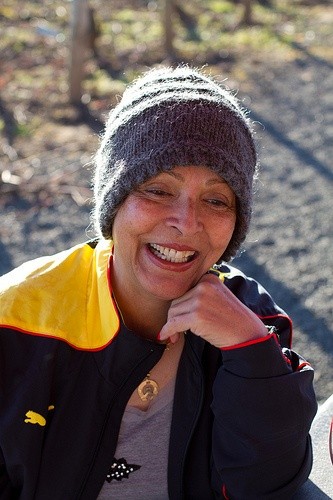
[92, 62, 256, 265]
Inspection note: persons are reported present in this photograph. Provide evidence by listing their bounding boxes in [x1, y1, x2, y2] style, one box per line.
[0, 60, 318, 500]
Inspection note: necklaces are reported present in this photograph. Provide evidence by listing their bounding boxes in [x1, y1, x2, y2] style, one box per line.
[111, 286, 173, 404]
